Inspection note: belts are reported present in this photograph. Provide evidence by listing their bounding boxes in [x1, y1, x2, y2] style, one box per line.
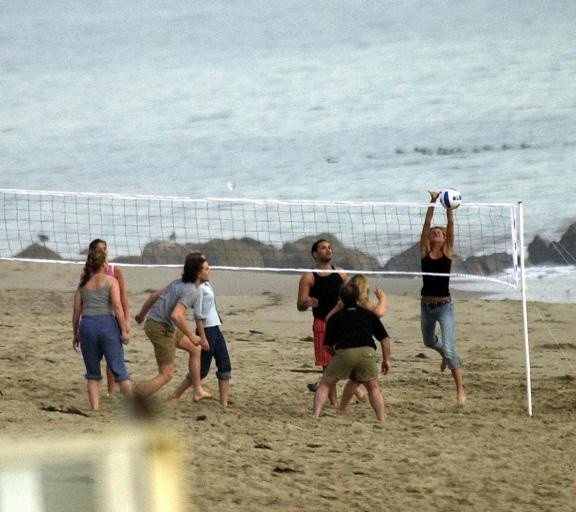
[422, 299, 448, 310]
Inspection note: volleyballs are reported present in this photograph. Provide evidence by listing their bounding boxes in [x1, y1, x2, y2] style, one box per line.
[440, 187, 462, 210]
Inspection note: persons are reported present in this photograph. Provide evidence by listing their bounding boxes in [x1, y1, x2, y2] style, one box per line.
[87, 238, 131, 397]
[166, 250, 233, 407]
[133, 255, 220, 417]
[296, 238, 369, 410]
[419, 189, 466, 408]
[306, 282, 392, 424]
[71, 246, 134, 411]
[325, 274, 388, 414]
